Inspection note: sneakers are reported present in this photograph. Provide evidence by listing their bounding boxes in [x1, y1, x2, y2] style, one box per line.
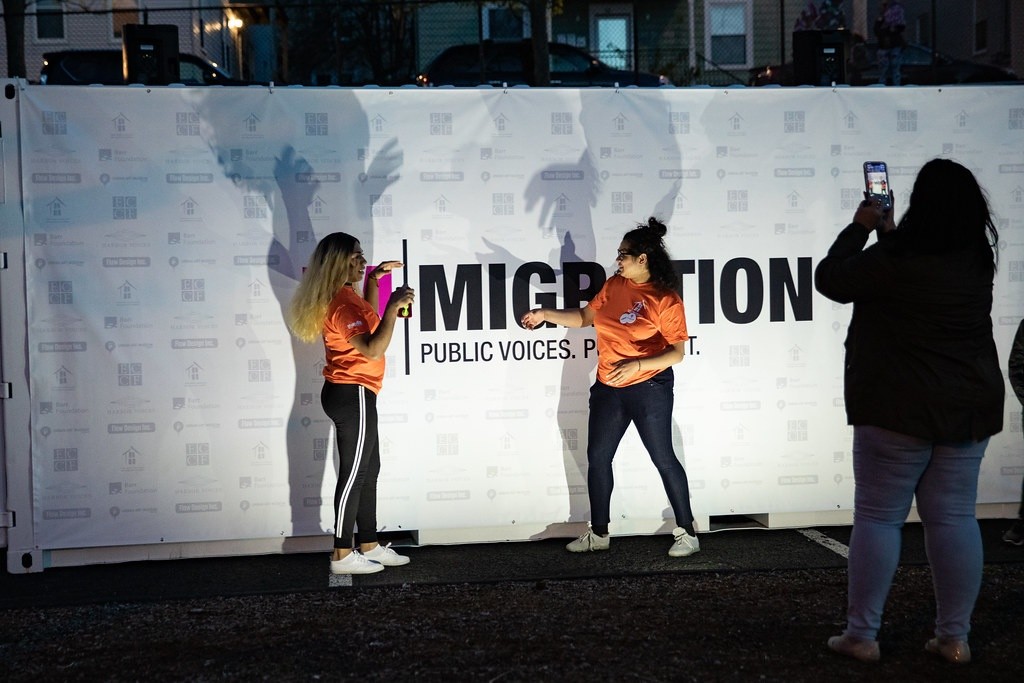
[330, 550, 384, 575]
[360, 542, 410, 566]
[668, 527, 700, 557]
[566, 530, 609, 552]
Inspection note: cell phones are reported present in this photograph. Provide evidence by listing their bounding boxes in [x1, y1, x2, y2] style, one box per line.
[863, 161, 892, 209]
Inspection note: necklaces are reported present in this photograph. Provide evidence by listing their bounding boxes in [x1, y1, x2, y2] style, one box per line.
[345, 282, 357, 292]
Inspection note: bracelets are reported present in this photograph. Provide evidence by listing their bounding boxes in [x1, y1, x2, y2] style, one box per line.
[637, 359, 640, 372]
[368, 274, 380, 288]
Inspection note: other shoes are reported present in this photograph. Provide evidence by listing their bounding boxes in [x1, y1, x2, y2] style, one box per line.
[925, 638, 970, 664]
[827, 630, 879, 661]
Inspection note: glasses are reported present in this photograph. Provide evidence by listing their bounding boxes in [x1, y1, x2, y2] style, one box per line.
[618, 249, 631, 260]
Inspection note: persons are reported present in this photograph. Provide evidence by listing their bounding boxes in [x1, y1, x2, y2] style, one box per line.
[1004, 319, 1024, 545]
[814, 159, 1006, 663]
[520, 217, 700, 557]
[868, 178, 887, 194]
[288, 232, 415, 574]
[875, 0, 908, 86]
